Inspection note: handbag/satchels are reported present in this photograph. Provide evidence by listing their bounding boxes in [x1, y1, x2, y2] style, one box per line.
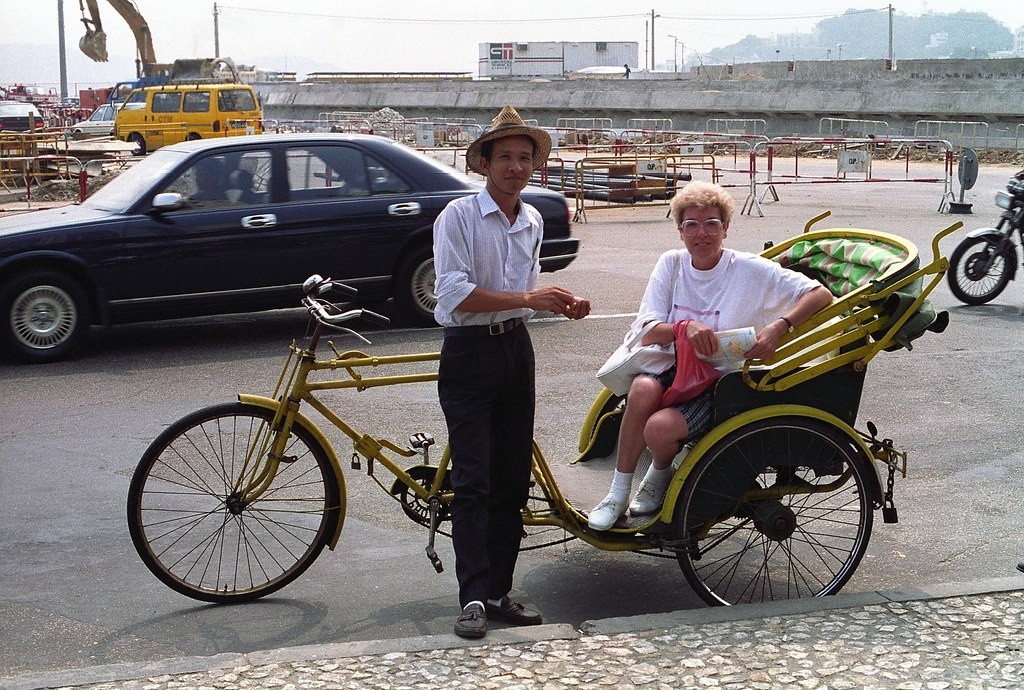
[596, 321, 675, 397]
[657, 320, 720, 409]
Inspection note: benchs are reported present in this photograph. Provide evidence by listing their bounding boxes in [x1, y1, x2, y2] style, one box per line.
[616, 296, 870, 482]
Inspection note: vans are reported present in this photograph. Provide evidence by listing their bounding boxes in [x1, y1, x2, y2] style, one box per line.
[108, 79, 263, 157]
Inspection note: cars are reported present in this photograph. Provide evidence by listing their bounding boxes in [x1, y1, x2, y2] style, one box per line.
[0, 102, 45, 141]
[0, 132, 585, 364]
[69, 101, 147, 137]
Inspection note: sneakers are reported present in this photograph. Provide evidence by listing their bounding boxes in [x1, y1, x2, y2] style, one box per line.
[629, 470, 676, 515]
[588, 496, 629, 530]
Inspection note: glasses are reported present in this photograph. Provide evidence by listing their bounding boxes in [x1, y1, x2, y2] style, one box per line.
[680, 219, 725, 235]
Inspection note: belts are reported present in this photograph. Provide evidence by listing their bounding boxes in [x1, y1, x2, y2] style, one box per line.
[444, 317, 524, 336]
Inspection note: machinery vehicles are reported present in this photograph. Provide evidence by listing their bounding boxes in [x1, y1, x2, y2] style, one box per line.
[78, 0, 175, 83]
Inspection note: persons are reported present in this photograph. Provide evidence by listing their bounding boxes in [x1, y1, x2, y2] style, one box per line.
[588, 181, 833, 531]
[192, 158, 229, 201]
[331, 123, 343, 133]
[432, 105, 590, 638]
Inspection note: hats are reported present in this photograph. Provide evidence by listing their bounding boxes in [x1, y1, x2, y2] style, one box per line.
[466, 105, 552, 175]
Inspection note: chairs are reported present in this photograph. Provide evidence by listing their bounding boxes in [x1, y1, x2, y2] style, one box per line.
[223, 171, 255, 206]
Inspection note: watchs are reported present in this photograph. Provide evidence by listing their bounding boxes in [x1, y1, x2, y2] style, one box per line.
[779, 317, 794, 333]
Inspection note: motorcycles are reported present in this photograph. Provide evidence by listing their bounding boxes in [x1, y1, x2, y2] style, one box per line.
[946, 173, 1024, 307]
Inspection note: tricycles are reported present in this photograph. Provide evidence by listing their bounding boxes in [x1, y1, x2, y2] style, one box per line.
[127, 206, 963, 610]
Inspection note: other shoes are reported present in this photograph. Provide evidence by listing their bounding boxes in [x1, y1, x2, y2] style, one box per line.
[486, 595, 542, 626]
[454, 603, 487, 637]
